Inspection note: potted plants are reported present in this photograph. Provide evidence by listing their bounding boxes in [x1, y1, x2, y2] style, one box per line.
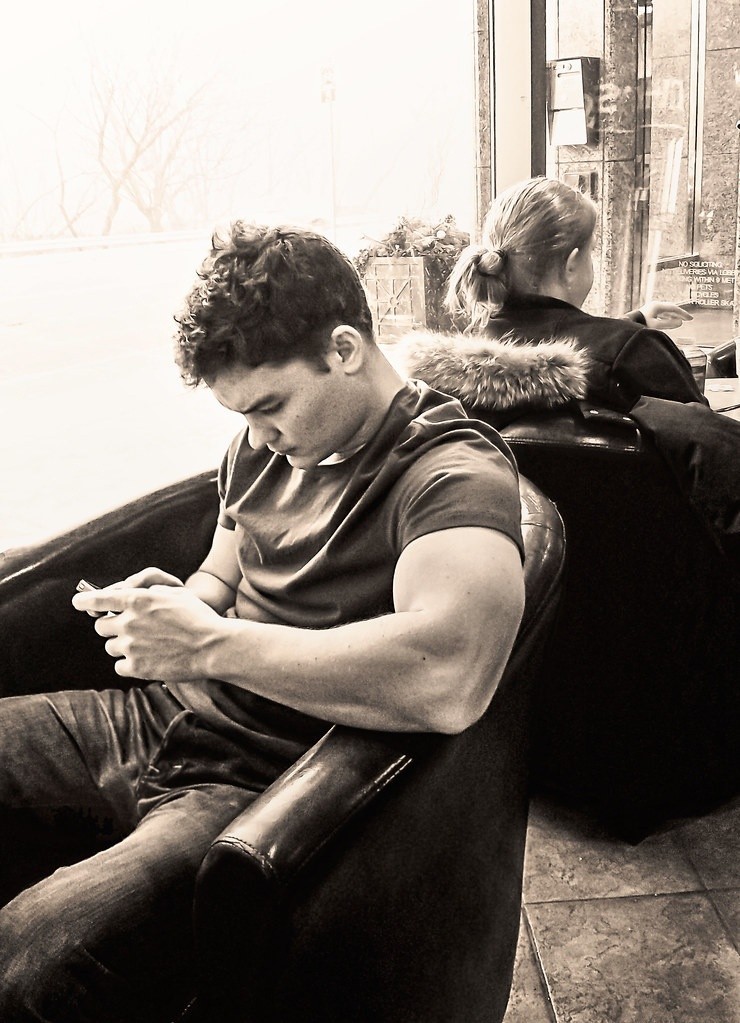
[351, 215, 474, 341]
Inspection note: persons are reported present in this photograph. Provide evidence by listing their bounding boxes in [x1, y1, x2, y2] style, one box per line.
[451, 178, 711, 412]
[0, 219, 525, 1023]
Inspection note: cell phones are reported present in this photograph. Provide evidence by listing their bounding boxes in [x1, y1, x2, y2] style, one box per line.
[77, 579, 119, 618]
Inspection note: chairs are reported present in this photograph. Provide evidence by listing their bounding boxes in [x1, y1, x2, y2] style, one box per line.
[493, 401, 740, 842]
[0, 467, 565, 1023]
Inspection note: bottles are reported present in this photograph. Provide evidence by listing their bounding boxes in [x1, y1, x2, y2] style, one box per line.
[673, 338, 707, 395]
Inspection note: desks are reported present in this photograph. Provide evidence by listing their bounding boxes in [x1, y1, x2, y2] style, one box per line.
[701, 377, 740, 420]
